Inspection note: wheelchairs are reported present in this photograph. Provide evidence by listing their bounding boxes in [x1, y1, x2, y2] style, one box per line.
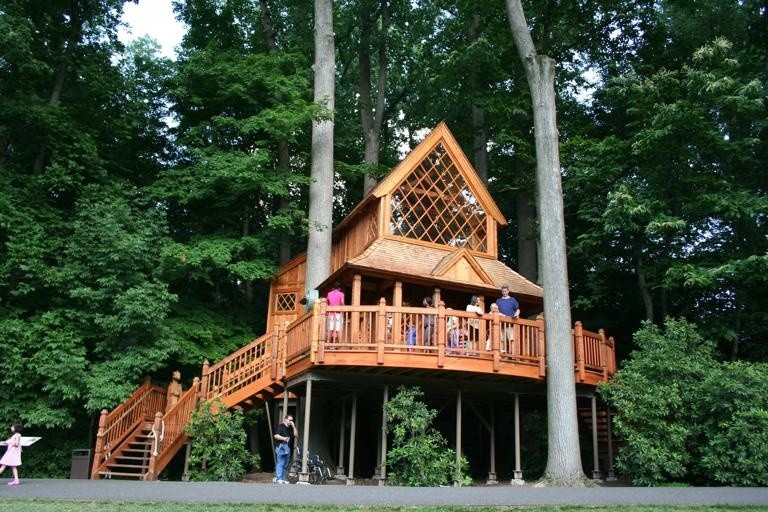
[286, 446, 336, 484]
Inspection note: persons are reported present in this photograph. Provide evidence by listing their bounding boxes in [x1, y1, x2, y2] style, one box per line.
[0, 421, 23, 484]
[327, 282, 346, 343]
[273, 415, 298, 484]
[383, 283, 522, 362]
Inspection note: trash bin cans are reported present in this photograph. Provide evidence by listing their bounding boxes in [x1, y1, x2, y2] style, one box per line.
[70, 449, 92, 479]
